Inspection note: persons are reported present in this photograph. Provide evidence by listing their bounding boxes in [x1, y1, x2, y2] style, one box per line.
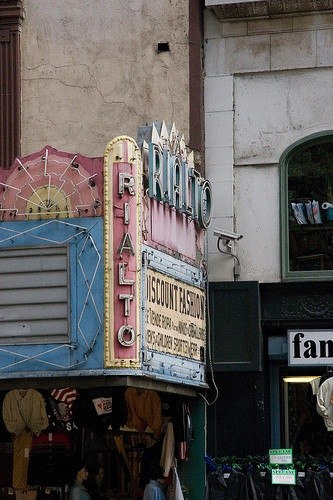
[66, 459, 92, 499]
[143, 467, 166, 500]
[88, 461, 109, 499]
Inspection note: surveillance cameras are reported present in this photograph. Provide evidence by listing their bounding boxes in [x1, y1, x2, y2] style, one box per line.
[214, 228, 243, 242]
